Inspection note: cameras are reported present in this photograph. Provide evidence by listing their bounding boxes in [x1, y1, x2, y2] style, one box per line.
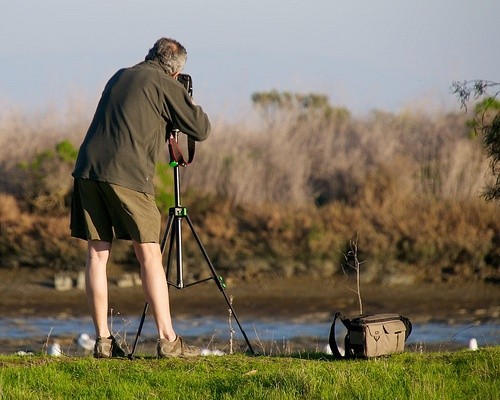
[177, 73, 190, 92]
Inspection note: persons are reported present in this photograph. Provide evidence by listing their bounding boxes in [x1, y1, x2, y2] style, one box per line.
[67, 36, 212, 361]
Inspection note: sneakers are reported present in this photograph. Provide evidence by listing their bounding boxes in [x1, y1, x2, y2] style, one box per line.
[156, 334, 200, 360]
[93, 335, 125, 359]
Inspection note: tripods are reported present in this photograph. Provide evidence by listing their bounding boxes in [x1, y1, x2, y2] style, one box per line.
[123, 126, 261, 361]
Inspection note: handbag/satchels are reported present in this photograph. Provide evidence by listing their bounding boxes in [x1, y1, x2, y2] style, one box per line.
[328, 312, 412, 359]
